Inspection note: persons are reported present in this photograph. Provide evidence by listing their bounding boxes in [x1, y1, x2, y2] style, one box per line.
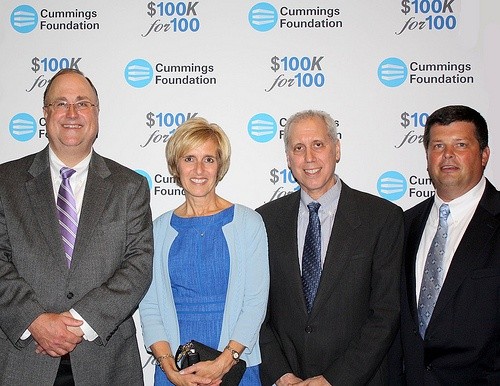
[400, 105, 500, 386]
[0, 69, 154, 386]
[138, 117, 270, 386]
[255, 110, 404, 386]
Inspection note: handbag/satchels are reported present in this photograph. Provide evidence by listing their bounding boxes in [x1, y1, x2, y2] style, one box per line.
[174, 340, 246, 386]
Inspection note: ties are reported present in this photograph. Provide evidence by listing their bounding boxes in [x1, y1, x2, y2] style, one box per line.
[303, 202, 322, 315]
[56, 167, 78, 269]
[418, 203, 450, 341]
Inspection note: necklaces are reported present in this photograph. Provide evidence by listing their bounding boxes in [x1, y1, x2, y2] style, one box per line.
[190, 216, 212, 237]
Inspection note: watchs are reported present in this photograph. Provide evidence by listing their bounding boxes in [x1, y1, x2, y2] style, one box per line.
[223, 346, 239, 364]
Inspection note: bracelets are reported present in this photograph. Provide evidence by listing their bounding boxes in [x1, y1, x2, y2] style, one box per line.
[156, 354, 175, 372]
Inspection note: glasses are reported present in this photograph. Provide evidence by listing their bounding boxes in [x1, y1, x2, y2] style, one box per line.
[43, 101, 98, 113]
